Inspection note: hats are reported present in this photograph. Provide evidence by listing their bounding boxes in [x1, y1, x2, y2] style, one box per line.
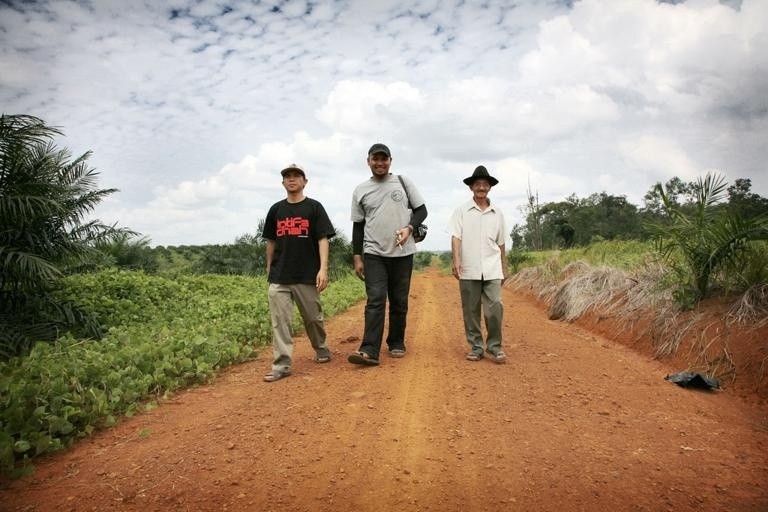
[281, 163, 305, 178]
[463, 166, 498, 186]
[369, 144, 390, 157]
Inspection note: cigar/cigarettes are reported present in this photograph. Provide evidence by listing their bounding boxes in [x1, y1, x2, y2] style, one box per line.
[396, 238, 404, 251]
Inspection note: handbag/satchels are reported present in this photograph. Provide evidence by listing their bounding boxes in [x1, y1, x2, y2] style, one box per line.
[413, 223, 427, 243]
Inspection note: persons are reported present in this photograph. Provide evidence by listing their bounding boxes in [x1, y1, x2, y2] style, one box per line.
[451, 166, 512, 362]
[263, 162, 337, 382]
[348, 144, 428, 366]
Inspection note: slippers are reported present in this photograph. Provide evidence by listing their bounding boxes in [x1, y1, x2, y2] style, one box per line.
[316, 350, 331, 363]
[348, 348, 405, 365]
[263, 370, 290, 381]
[466, 352, 506, 361]
